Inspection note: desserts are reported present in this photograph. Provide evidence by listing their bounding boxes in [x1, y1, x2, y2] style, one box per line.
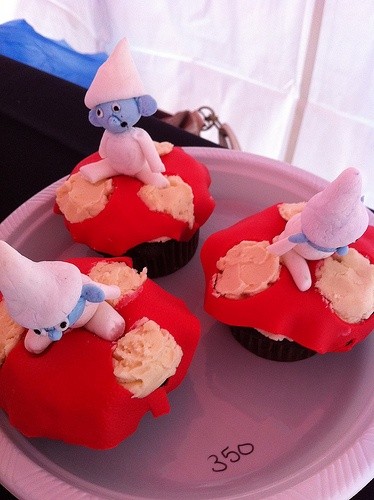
[0, 240, 202, 452]
[200, 168, 374, 362]
[52, 39, 216, 279]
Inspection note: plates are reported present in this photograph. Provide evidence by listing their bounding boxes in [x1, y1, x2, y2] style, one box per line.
[1, 147, 374, 500]
